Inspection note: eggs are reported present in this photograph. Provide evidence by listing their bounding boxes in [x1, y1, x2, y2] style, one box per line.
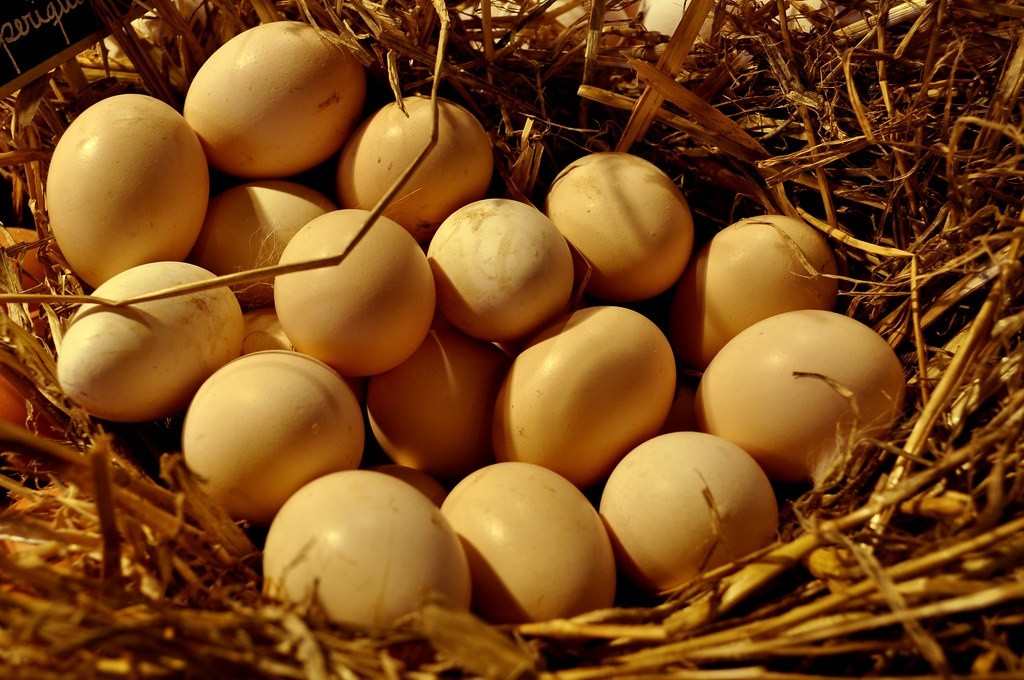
[0, 21, 904, 634]
